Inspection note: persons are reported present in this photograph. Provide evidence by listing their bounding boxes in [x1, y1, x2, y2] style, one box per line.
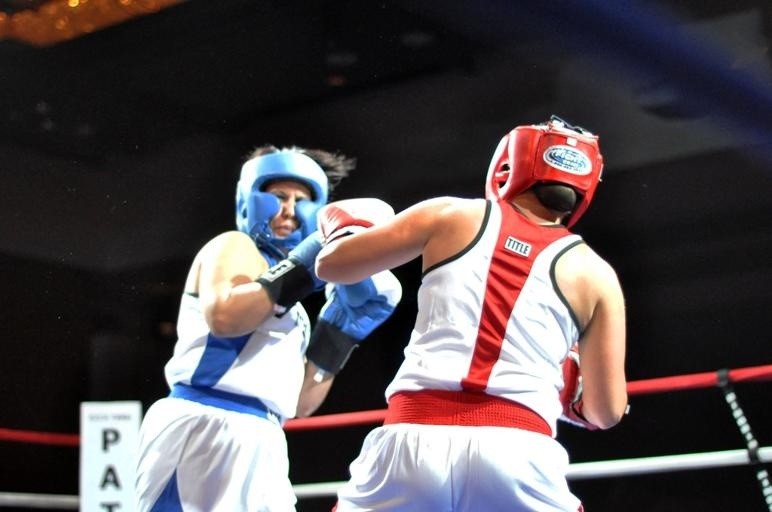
[126, 142, 403, 512]
[315, 116, 628, 512]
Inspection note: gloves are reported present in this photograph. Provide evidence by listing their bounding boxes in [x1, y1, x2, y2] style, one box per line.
[308, 269, 402, 372]
[256, 196, 397, 319]
[559, 342, 598, 431]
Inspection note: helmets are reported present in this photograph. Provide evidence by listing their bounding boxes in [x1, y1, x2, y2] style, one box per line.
[235, 150, 328, 246]
[485, 124, 603, 230]
